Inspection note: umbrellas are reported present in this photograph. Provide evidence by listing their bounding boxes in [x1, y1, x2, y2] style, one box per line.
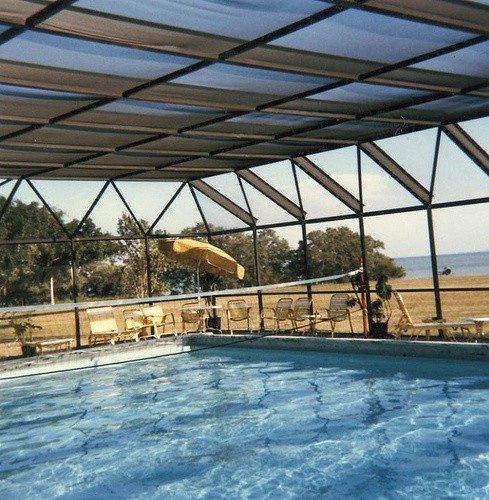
[158, 237, 245, 333]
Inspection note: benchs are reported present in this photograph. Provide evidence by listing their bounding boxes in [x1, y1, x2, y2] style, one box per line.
[38, 338, 74, 356]
[409, 322, 475, 344]
[467, 318, 489, 339]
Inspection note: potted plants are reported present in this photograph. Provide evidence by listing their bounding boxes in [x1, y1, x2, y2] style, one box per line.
[0, 310, 42, 357]
[201, 272, 221, 333]
[347, 274, 393, 338]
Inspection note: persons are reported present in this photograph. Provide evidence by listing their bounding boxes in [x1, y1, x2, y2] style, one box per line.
[444, 266, 453, 276]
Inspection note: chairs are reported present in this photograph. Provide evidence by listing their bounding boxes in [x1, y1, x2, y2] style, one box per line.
[86, 305, 177, 347]
[257, 293, 354, 337]
[226, 299, 252, 335]
[181, 303, 204, 333]
[393, 293, 429, 341]
